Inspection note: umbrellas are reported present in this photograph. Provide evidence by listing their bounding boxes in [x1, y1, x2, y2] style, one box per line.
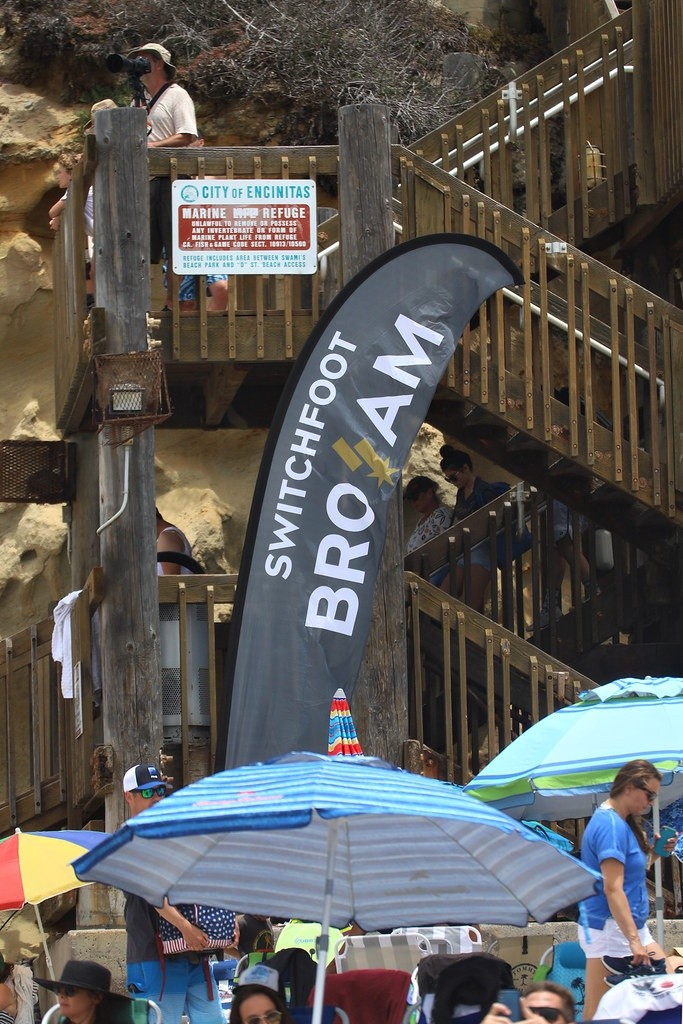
[0, 827, 111, 1000]
[325, 686, 369, 756]
[67, 752, 605, 1024]
[464, 676, 683, 947]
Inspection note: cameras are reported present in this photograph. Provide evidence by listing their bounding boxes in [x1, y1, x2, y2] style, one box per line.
[105, 52, 152, 75]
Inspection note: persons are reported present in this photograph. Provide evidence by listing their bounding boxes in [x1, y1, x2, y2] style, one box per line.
[404, 443, 601, 697]
[129, 44, 197, 263]
[156, 505, 192, 574]
[577, 759, 678, 1020]
[0, 952, 18, 1024]
[480, 980, 575, 1024]
[234, 915, 276, 970]
[31, 960, 135, 1024]
[123, 764, 239, 1024]
[159, 243, 228, 310]
[230, 984, 293, 1024]
[48, 98, 119, 310]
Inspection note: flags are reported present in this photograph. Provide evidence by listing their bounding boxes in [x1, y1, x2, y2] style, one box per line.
[212, 232, 524, 775]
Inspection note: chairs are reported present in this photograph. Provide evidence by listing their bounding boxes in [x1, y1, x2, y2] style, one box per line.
[39, 924, 586, 1024]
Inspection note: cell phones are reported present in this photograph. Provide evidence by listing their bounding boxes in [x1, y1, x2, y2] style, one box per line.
[496, 989, 521, 1022]
[652, 825, 676, 858]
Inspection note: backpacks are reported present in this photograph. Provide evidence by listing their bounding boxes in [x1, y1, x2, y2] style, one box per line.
[154, 902, 236, 957]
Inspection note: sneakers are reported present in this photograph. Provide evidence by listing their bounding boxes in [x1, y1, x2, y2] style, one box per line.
[601, 951, 668, 988]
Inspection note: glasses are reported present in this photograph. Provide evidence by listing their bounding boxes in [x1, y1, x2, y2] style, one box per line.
[53, 985, 78, 997]
[638, 786, 657, 801]
[529, 1006, 568, 1024]
[247, 1011, 282, 1024]
[141, 786, 165, 798]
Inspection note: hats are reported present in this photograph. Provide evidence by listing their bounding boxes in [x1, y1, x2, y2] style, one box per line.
[122, 764, 174, 792]
[232, 964, 286, 999]
[32, 959, 136, 1001]
